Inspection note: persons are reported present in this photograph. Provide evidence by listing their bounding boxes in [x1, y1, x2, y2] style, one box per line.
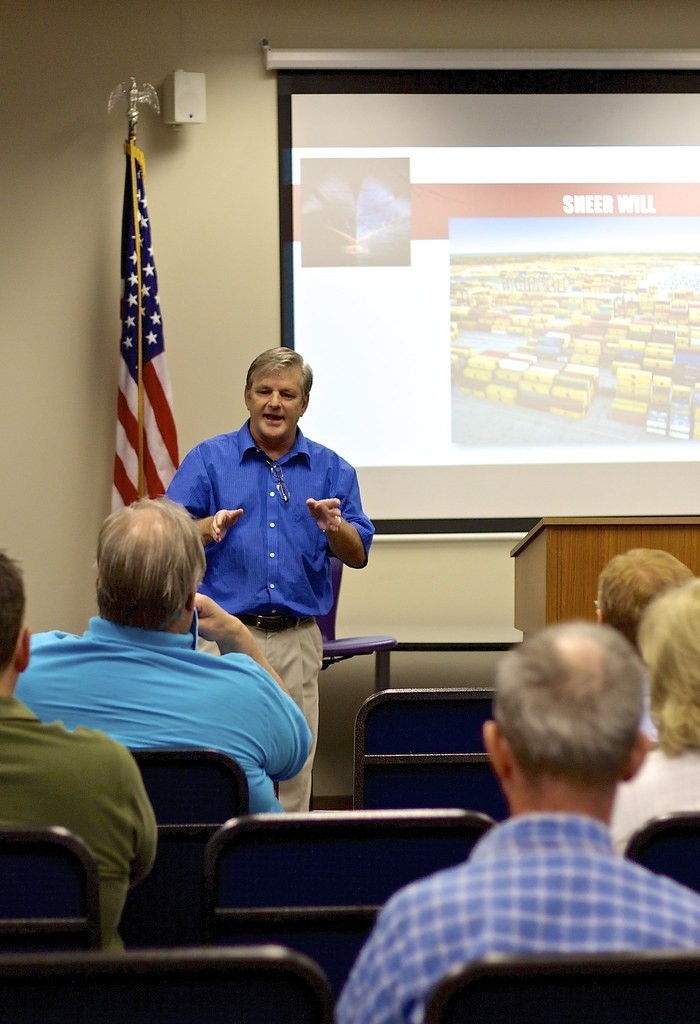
[0, 494, 312, 953]
[164, 348, 375, 806]
[332, 546, 700, 1024]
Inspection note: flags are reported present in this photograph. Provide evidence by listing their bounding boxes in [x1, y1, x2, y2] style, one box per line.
[108, 136, 179, 511]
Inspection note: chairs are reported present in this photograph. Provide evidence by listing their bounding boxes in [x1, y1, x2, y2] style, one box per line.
[0, 686, 700, 1024]
[314, 555, 397, 690]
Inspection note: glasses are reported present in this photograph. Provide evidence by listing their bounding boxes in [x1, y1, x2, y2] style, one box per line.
[268, 460, 291, 503]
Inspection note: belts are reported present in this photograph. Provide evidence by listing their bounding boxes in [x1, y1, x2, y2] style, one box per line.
[233, 612, 310, 631]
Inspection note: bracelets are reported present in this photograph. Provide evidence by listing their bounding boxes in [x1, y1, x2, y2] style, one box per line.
[332, 506, 341, 533]
